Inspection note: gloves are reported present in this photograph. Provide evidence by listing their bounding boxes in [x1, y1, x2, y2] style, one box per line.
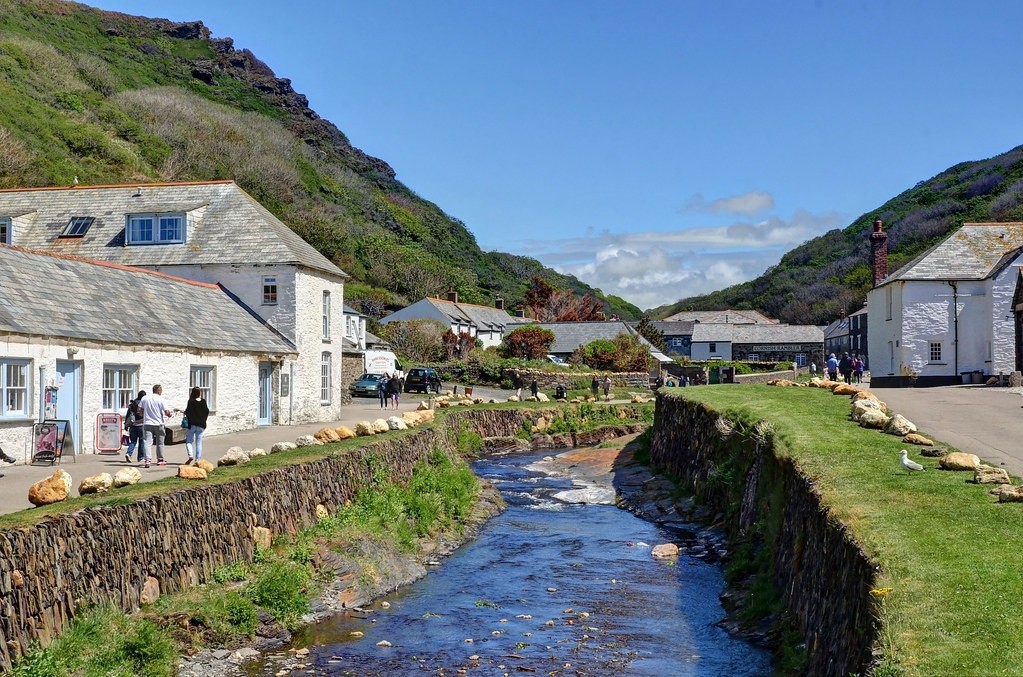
[124, 426, 128, 431]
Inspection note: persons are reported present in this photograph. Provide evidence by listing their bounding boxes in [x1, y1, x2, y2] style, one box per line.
[513, 373, 523, 396]
[173, 387, 210, 466]
[695, 374, 707, 385]
[0, 447, 16, 478]
[125, 391, 146, 463]
[679, 376, 686, 387]
[591, 377, 599, 402]
[839, 352, 863, 385]
[378, 372, 402, 410]
[666, 378, 675, 387]
[137, 385, 172, 467]
[531, 378, 537, 396]
[811, 362, 817, 377]
[827, 353, 837, 381]
[603, 375, 611, 401]
[655, 374, 664, 388]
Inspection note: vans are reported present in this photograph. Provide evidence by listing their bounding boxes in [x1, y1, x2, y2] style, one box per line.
[403, 367, 443, 395]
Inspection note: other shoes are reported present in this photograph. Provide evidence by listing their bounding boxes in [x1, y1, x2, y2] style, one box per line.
[4, 457, 16, 463]
[0, 474, 4, 477]
[145, 461, 150, 468]
[156, 459, 167, 465]
[126, 454, 131, 462]
[186, 457, 193, 465]
[138, 459, 145, 464]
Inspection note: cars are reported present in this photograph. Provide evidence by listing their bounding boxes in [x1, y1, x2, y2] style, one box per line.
[543, 354, 571, 367]
[348, 373, 394, 399]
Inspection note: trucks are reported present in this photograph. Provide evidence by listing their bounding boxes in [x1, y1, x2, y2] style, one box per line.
[361, 350, 405, 388]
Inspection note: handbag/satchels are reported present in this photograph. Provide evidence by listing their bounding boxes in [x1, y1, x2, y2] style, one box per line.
[181, 412, 192, 430]
[125, 406, 135, 427]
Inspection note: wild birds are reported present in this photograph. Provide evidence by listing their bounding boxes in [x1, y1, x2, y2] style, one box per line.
[72, 175, 79, 186]
[898, 450, 928, 475]
